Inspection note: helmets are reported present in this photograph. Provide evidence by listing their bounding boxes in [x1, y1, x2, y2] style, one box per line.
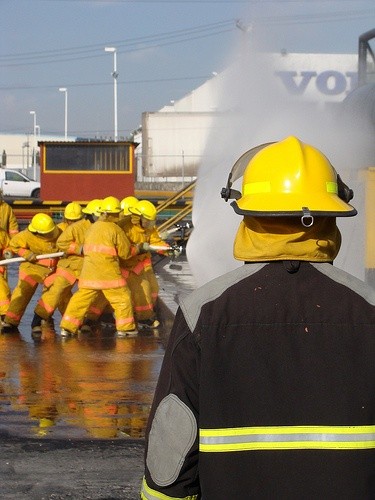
[120, 196, 139, 216]
[64, 203, 84, 220]
[230, 134, 358, 227]
[97, 196, 121, 213]
[28, 213, 55, 234]
[36, 419, 55, 429]
[128, 200, 156, 221]
[82, 199, 104, 217]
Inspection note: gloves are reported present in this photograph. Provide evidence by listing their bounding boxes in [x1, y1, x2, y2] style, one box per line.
[138, 243, 149, 255]
[57, 249, 69, 258]
[167, 246, 182, 259]
[18, 249, 36, 263]
[3, 251, 12, 259]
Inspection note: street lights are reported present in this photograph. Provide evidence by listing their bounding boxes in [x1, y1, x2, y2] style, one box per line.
[58, 88, 68, 140]
[35, 125, 40, 155]
[28, 110, 37, 181]
[103, 46, 118, 142]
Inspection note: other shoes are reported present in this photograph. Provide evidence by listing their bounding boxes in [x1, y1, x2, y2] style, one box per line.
[81, 319, 93, 332]
[1, 315, 7, 326]
[31, 333, 42, 344]
[153, 307, 161, 313]
[3, 322, 17, 332]
[96, 313, 116, 327]
[32, 318, 42, 333]
[136, 318, 160, 328]
[60, 327, 74, 336]
[117, 329, 138, 336]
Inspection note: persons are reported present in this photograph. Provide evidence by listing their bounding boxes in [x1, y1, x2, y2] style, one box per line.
[140, 137, 375, 500]
[0, 186, 181, 337]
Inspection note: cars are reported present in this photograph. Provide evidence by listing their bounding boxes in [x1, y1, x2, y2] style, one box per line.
[0, 169, 41, 198]
